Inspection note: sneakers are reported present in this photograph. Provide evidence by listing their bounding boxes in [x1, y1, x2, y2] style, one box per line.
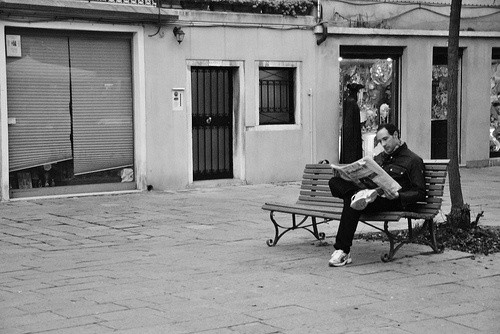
[328, 248, 352, 267]
[350, 188, 377, 211]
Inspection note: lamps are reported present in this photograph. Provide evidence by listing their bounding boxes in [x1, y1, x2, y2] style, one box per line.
[173, 25, 185, 45]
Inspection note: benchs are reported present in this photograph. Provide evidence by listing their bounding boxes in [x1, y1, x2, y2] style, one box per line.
[260, 159, 453, 263]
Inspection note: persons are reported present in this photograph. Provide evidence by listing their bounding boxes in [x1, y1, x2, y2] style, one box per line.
[328, 122, 427, 268]
